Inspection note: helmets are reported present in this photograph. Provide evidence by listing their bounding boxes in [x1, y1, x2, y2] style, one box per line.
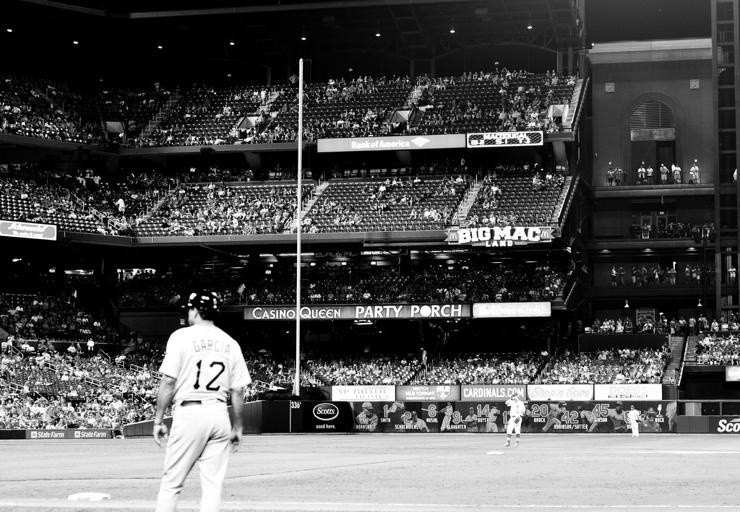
[187, 292, 220, 315]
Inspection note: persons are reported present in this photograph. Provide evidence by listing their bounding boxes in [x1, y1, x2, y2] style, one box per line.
[0, 65, 739, 438]
[150, 289, 253, 512]
[504, 390, 526, 448]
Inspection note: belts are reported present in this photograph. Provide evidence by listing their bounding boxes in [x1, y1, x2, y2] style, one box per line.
[181, 398, 223, 406]
